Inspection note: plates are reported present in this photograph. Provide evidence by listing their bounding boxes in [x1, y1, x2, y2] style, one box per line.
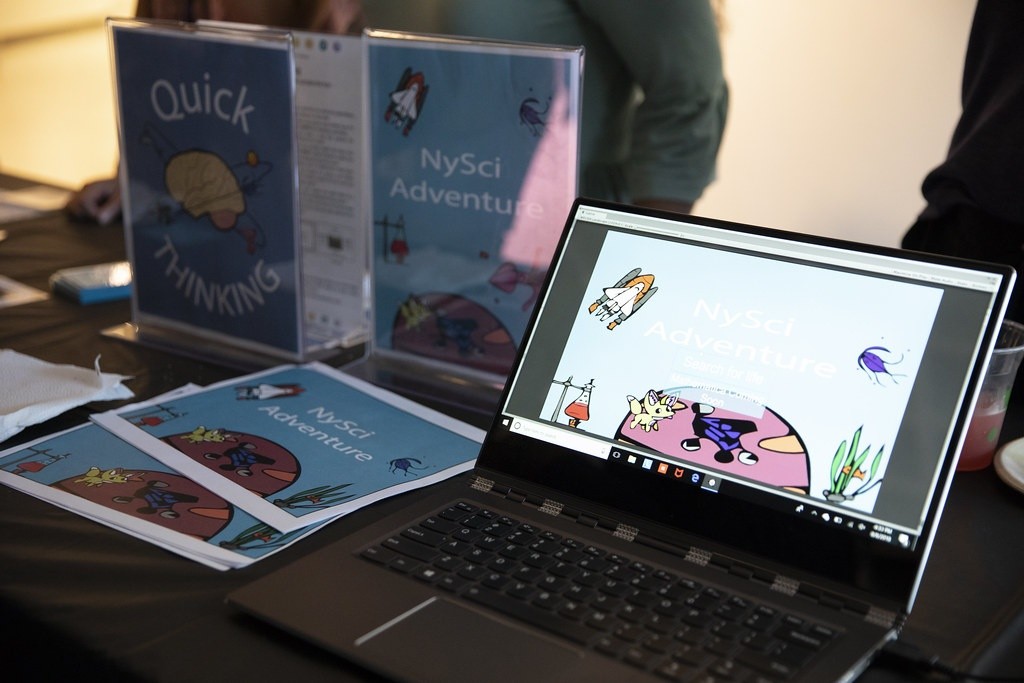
[993, 437, 1024, 493]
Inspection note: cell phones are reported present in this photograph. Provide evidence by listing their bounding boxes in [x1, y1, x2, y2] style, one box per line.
[49, 260, 132, 304]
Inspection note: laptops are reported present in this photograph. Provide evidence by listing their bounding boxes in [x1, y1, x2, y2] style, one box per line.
[224, 193, 1017, 682]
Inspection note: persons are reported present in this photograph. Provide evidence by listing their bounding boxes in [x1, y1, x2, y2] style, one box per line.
[67, 0, 729, 225]
[901, 0, 1024, 403]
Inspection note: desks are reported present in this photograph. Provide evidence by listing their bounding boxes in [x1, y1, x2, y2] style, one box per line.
[1, 174, 1024, 683]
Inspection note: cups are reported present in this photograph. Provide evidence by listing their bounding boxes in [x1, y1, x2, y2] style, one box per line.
[956, 319, 1024, 471]
